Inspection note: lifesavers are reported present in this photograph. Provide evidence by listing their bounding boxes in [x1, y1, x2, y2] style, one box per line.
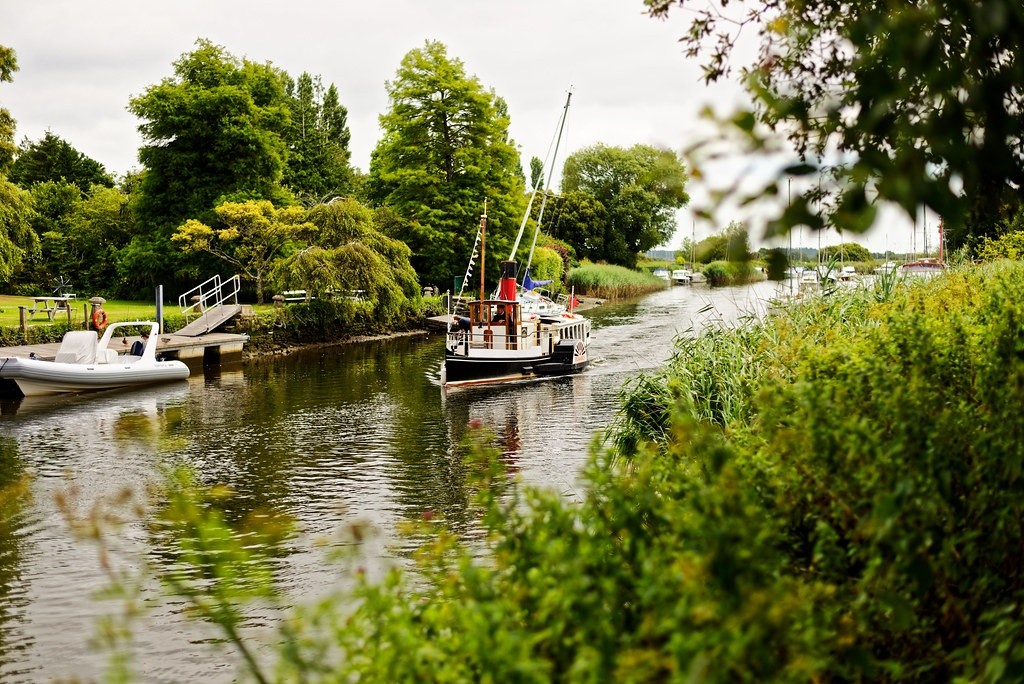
[94, 310, 107, 329]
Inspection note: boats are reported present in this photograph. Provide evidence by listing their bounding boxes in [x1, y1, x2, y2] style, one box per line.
[672, 270, 691, 285]
[690, 272, 708, 283]
[798, 270, 819, 292]
[795, 266, 808, 277]
[0, 321, 190, 396]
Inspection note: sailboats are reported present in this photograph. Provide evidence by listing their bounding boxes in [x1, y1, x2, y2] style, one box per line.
[685, 220, 696, 274]
[903, 200, 950, 272]
[440, 85, 592, 387]
[839, 226, 857, 281]
[873, 235, 900, 277]
[786, 176, 798, 278]
[653, 269, 670, 288]
[815, 176, 827, 274]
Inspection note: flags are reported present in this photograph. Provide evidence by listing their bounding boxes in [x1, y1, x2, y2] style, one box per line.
[570, 296, 579, 310]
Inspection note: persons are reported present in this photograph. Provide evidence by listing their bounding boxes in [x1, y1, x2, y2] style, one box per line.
[492, 305, 513, 327]
[140, 333, 149, 352]
[454, 317, 470, 328]
